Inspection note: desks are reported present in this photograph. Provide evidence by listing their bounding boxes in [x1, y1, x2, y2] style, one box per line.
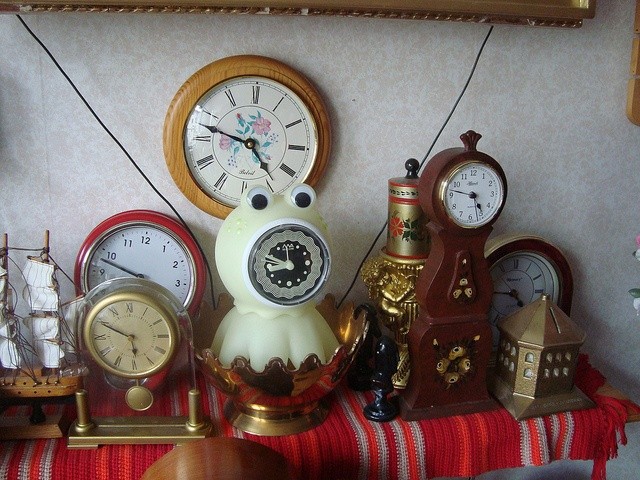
[0, 353, 18, 479]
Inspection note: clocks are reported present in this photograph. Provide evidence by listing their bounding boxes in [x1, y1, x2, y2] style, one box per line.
[69, 278, 212, 446]
[488, 234, 574, 346]
[162, 55, 334, 221]
[210, 186, 341, 371]
[394, 130, 506, 416]
[73, 211, 206, 317]
